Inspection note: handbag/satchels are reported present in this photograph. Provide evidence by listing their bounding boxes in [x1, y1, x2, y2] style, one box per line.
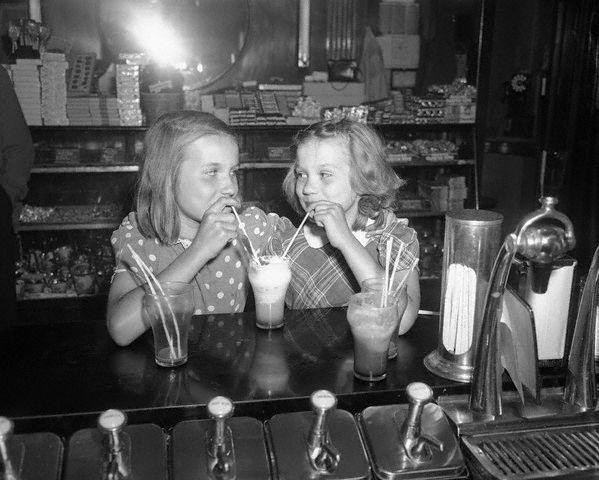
[327, 58, 364, 91]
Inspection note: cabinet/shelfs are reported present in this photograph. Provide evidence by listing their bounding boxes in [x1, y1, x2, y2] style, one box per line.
[0, 118, 479, 299]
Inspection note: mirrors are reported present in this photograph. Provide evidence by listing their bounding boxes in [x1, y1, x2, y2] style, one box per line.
[96, 0, 250, 94]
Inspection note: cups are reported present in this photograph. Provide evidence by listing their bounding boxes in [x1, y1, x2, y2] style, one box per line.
[143, 282, 195, 366]
[248, 258, 292, 331]
[346, 279, 409, 382]
[424, 210, 503, 385]
[71, 264, 95, 296]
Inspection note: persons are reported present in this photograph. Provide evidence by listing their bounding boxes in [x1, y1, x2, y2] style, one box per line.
[263, 117, 423, 335]
[105, 108, 294, 348]
